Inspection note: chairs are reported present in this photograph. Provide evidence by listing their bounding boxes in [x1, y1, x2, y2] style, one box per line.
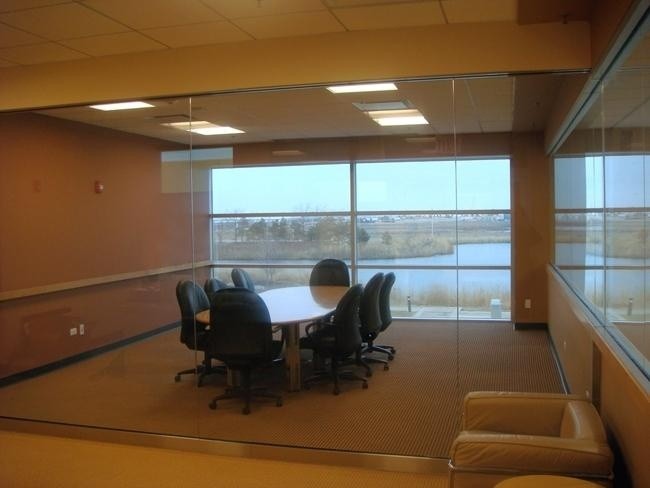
[449, 391, 615, 488]
[176, 259, 396, 415]
